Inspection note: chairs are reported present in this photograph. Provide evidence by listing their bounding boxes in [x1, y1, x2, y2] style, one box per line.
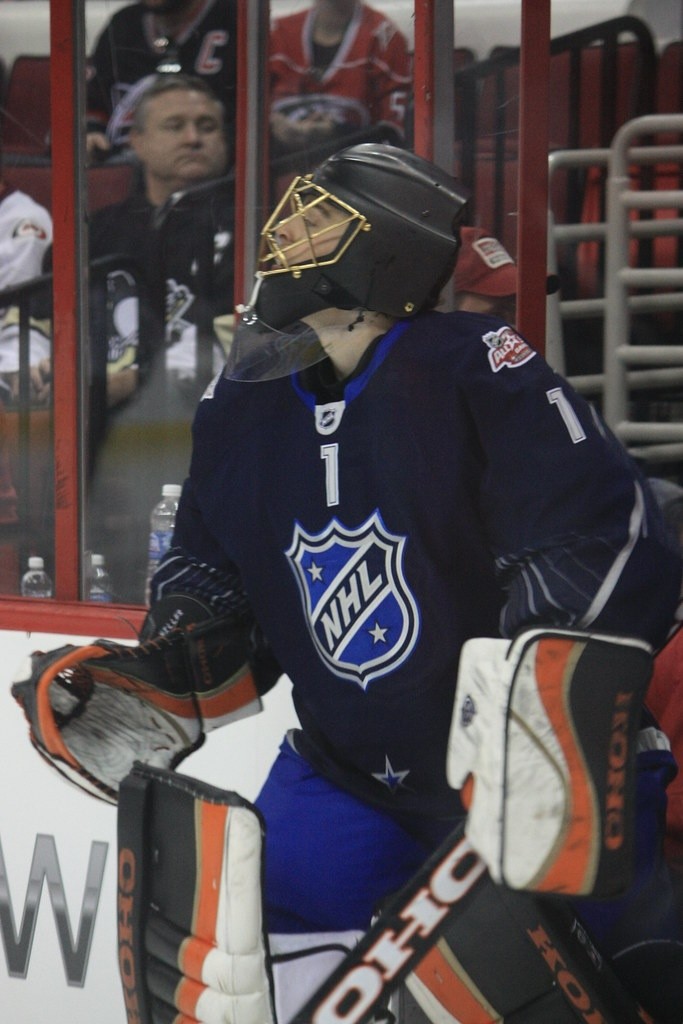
[1, 40, 683, 299]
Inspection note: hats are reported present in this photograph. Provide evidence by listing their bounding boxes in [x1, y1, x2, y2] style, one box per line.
[454, 224, 518, 297]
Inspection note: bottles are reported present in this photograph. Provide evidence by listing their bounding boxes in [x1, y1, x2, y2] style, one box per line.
[144, 483, 184, 609]
[21, 556, 53, 600]
[90, 554, 113, 603]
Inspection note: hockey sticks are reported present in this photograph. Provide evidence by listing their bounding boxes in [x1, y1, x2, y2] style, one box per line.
[287, 816, 491, 1024]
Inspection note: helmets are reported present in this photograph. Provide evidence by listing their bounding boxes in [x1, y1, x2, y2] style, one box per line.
[241, 144, 469, 332]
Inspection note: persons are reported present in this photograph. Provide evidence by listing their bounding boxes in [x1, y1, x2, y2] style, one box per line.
[6, 140, 683, 1023]
[0, 0, 560, 604]
